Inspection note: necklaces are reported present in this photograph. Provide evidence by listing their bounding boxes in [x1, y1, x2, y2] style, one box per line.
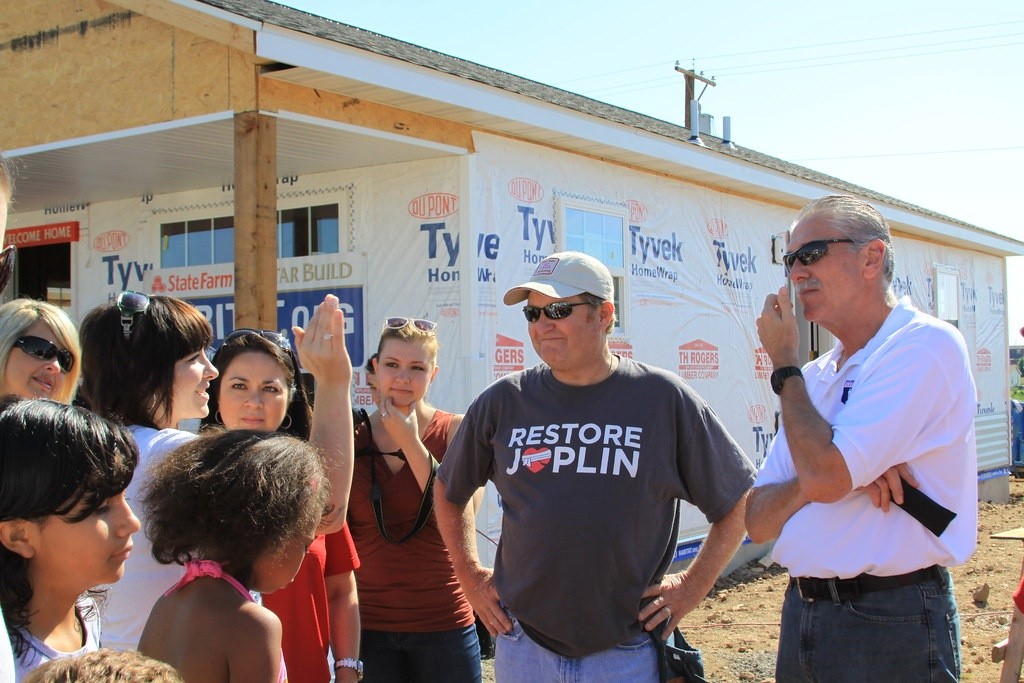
[837, 351, 844, 372]
[608, 355, 614, 378]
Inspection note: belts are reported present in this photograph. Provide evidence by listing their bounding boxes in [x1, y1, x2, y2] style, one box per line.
[787, 566, 934, 602]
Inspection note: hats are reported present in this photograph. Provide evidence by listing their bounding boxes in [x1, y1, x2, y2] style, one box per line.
[503, 251, 615, 304]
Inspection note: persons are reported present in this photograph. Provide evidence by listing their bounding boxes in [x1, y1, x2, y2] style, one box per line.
[744, 194, 980, 683]
[0, 156, 483, 683]
[434, 251, 758, 683]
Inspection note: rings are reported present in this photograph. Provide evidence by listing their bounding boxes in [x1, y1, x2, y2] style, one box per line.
[322, 333, 332, 340]
[665, 606, 673, 616]
[382, 412, 389, 417]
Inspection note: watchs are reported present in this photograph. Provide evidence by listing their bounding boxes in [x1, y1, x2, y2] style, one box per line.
[334, 658, 364, 682]
[770, 366, 805, 394]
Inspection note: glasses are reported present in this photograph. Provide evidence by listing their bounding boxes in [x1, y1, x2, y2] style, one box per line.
[522, 302, 602, 323]
[13, 335, 75, 374]
[215, 328, 292, 367]
[117, 289, 151, 340]
[381, 316, 438, 337]
[782, 238, 865, 274]
